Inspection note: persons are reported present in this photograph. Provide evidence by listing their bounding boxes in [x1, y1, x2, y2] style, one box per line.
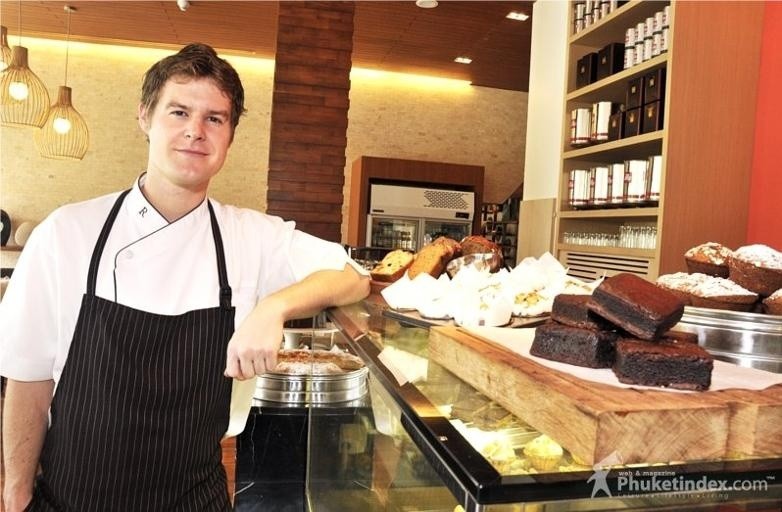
[1, 39, 376, 512]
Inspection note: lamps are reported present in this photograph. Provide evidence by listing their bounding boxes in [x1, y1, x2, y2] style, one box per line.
[0, 1, 91, 162]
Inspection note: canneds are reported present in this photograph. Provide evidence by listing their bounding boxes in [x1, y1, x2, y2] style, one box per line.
[573, 1, 670, 69]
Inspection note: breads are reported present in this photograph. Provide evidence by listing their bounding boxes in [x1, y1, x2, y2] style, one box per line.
[277, 349, 364, 370]
[370, 236, 710, 391]
[276, 363, 342, 374]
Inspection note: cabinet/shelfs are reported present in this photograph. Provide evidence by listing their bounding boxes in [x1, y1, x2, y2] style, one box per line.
[304, 296, 781, 512]
[552, 1, 766, 285]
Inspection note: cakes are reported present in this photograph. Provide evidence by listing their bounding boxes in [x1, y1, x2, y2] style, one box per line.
[655, 243, 782, 315]
[450, 395, 591, 475]
